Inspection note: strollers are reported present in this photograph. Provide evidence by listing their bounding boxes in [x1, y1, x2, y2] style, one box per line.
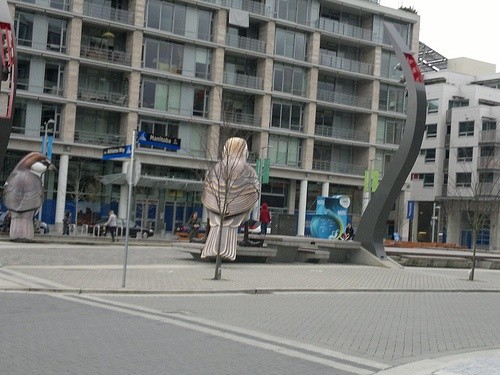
[339, 234, 350, 240]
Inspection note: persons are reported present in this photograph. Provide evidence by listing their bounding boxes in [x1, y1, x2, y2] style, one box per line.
[103, 210, 116, 242]
[189, 211, 201, 240]
[62, 211, 71, 235]
[346, 223, 354, 240]
[0, 212, 47, 233]
[260, 203, 271, 234]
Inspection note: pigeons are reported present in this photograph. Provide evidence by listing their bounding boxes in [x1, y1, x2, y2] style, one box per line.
[200, 137, 260, 262]
[2, 152, 55, 240]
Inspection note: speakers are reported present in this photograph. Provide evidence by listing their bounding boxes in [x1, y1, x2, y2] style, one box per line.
[271, 213, 298, 236]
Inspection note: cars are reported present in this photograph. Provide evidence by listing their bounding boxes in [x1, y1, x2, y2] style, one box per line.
[1, 213, 49, 233]
[240, 219, 271, 234]
[175, 222, 208, 238]
[82, 217, 153, 238]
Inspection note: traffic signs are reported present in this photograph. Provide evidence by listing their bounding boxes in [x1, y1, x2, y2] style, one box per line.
[102, 145, 132, 160]
